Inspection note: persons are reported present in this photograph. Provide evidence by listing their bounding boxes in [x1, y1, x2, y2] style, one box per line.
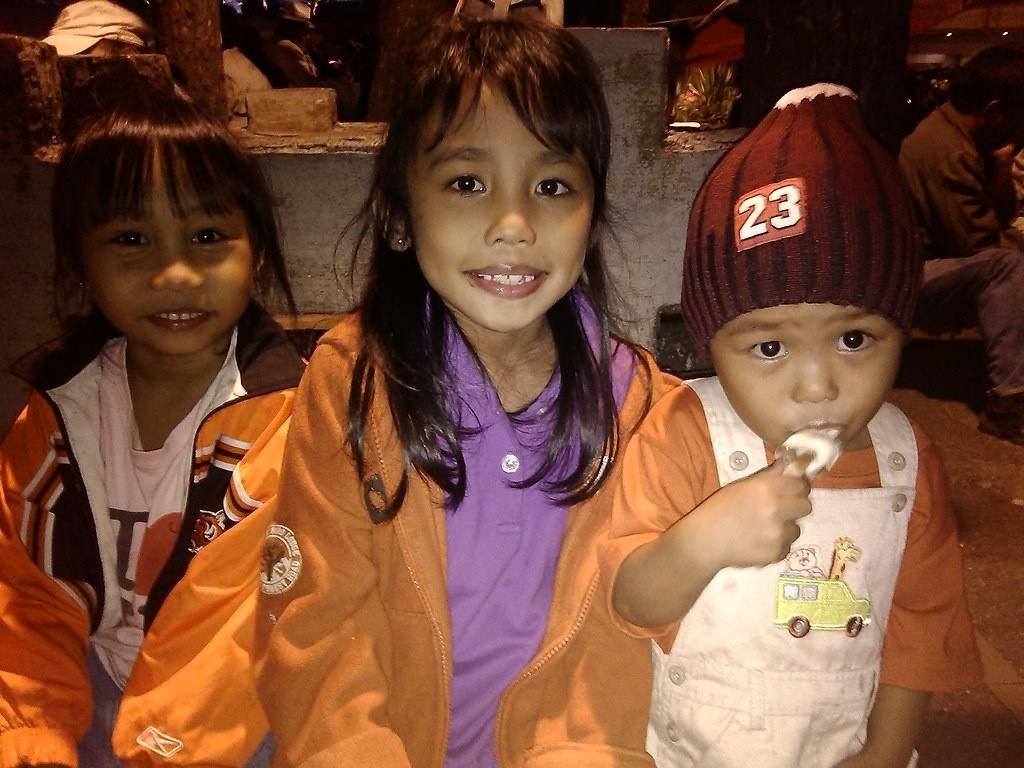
[598, 81, 984, 768]
[0, 95, 306, 767]
[897, 38, 1024, 440]
[220, 0, 561, 130]
[255, 12, 690, 768]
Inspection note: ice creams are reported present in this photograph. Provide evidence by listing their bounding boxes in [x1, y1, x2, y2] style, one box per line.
[776, 426, 841, 480]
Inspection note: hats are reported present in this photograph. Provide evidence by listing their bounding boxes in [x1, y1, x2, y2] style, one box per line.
[41, 0, 151, 56]
[679, 81, 926, 357]
